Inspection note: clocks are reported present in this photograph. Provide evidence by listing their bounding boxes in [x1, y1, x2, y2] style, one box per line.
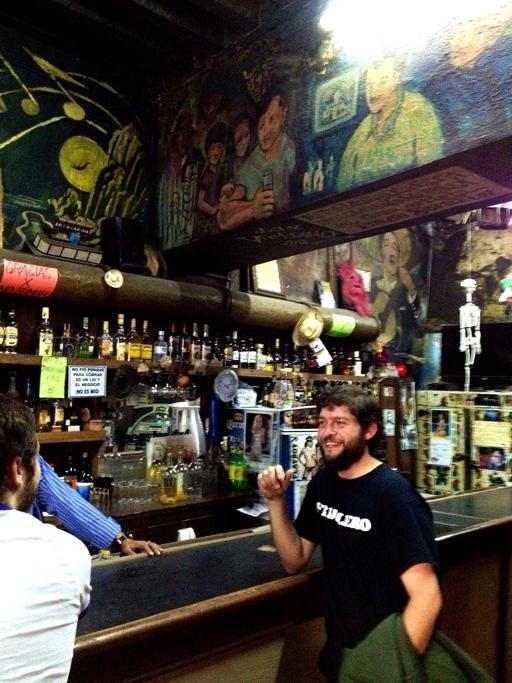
[213, 368, 239, 402]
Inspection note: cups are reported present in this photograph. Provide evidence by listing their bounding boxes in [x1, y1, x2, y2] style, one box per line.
[262, 171, 274, 200]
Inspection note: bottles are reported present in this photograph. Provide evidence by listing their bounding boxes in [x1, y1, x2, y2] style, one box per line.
[0, 306, 364, 377]
[3, 370, 381, 519]
[304, 154, 338, 195]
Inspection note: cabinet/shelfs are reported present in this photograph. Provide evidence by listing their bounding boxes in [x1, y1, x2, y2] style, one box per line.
[1, 247, 382, 488]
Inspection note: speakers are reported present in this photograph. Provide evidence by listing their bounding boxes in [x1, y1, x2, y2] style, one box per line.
[101, 216, 144, 274]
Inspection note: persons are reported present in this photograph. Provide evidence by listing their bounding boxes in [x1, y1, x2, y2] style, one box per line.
[257, 382, 443, 681]
[249, 415, 266, 462]
[297, 435, 320, 481]
[1, 388, 94, 681]
[27, 441, 164, 557]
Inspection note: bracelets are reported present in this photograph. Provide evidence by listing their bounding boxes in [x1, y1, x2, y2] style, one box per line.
[111, 529, 134, 553]
